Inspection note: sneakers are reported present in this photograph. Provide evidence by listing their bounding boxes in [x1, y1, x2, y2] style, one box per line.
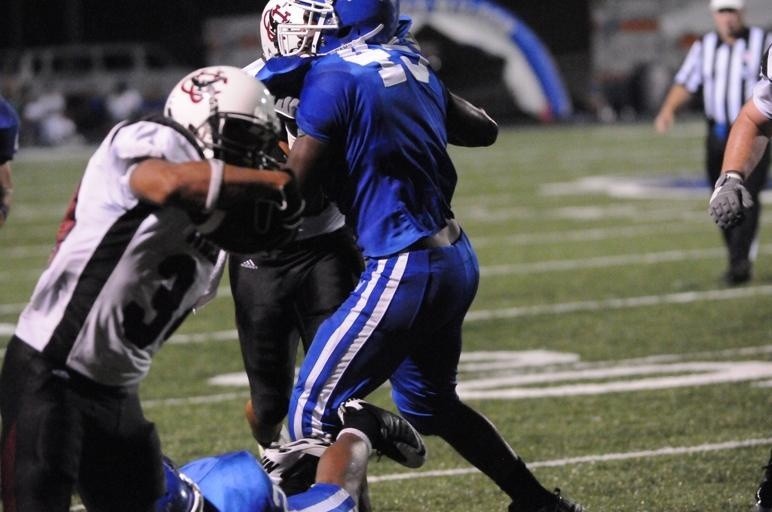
[337, 398, 426, 468]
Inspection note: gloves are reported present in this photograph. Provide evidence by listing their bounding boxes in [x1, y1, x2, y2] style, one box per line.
[708, 169, 753, 230]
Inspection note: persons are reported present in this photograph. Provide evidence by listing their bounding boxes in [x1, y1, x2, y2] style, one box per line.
[654, 1, 772, 285]
[158, 398, 426, 511]
[108, 82, 140, 119]
[228, 1, 365, 479]
[0, 96, 21, 225]
[0, 66, 307, 511]
[275, 0, 579, 512]
[29, 83, 76, 142]
[708, 34, 772, 232]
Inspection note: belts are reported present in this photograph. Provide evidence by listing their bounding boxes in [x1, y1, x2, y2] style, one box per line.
[398, 219, 460, 253]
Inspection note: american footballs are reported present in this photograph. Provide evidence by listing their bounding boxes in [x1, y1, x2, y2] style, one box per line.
[277, 142, 325, 215]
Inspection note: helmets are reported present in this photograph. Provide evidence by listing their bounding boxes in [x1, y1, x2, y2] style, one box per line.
[156, 454, 205, 512]
[260, 0, 400, 61]
[163, 65, 281, 159]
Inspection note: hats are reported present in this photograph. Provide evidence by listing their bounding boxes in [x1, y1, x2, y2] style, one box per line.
[710, 0, 744, 11]
